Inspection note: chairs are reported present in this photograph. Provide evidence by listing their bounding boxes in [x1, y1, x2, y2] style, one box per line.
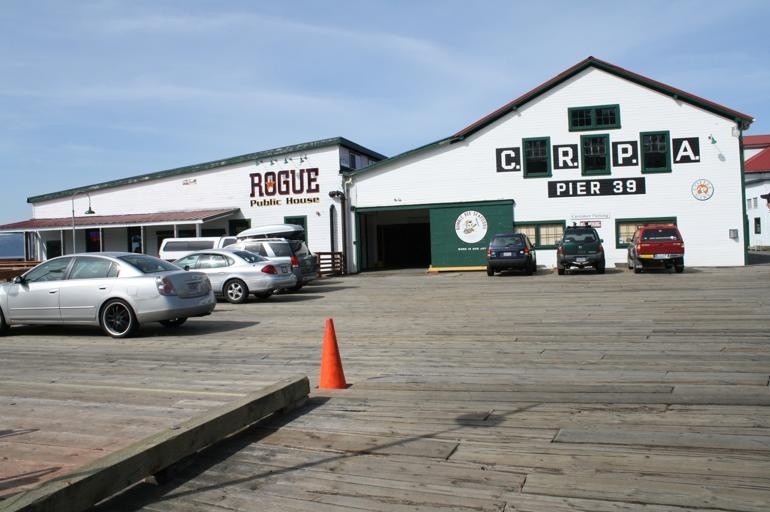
[584, 237, 593, 240]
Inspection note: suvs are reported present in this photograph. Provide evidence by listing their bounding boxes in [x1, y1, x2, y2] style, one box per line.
[628, 223, 685, 272]
[558, 226, 605, 274]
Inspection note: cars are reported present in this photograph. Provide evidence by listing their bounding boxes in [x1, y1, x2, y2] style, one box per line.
[157, 223, 320, 304]
[0, 252, 217, 337]
[487, 234, 536, 275]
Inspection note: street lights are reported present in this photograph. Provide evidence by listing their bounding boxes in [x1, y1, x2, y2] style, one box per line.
[72, 190, 94, 254]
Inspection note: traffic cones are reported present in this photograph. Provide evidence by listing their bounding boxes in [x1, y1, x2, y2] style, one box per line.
[318, 319, 350, 389]
